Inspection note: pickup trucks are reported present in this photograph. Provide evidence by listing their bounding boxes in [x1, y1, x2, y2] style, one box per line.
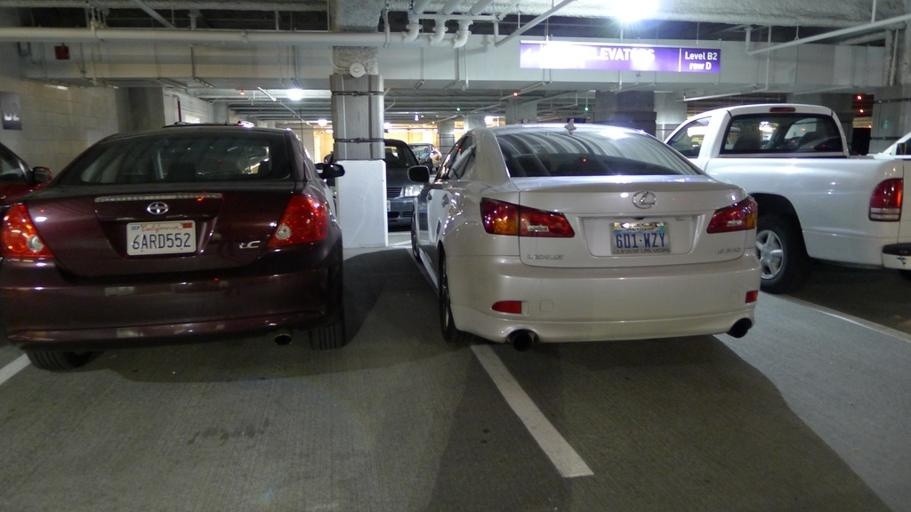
[665, 101, 911, 295]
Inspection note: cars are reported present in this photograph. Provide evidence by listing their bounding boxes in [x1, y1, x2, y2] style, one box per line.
[325, 140, 445, 228]
[881, 128, 911, 157]
[759, 122, 801, 143]
[408, 121, 763, 354]
[685, 125, 742, 151]
[1, 121, 347, 373]
[0, 143, 52, 199]
